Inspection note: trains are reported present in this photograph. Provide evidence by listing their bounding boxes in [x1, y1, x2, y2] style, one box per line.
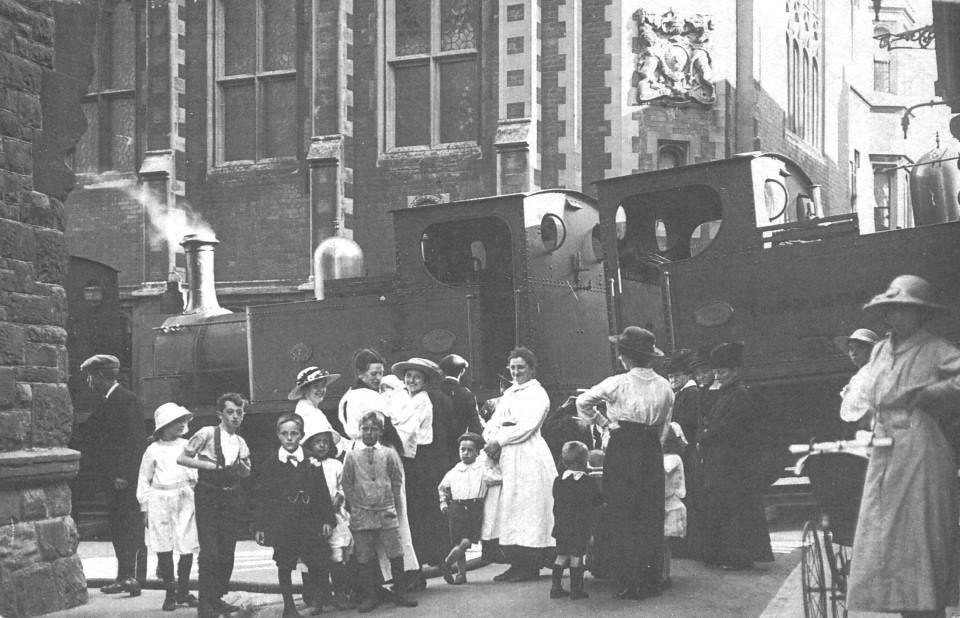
[138, 149, 960, 549]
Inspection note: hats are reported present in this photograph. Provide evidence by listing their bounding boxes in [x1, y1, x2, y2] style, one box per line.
[608, 326, 744, 375]
[457, 432, 485, 449]
[287, 366, 341, 400]
[300, 419, 341, 445]
[390, 358, 442, 384]
[862, 274, 953, 318]
[154, 402, 193, 432]
[79, 354, 120, 374]
[439, 354, 469, 374]
[833, 329, 879, 354]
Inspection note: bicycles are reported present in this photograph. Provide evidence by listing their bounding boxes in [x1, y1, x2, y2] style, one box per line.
[794, 435, 852, 618]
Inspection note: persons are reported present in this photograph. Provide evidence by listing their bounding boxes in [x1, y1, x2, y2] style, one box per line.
[840, 274, 960, 618]
[80, 325, 774, 618]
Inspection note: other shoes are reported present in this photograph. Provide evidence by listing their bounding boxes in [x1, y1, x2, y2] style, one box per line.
[175, 592, 199, 607]
[438, 563, 454, 583]
[162, 594, 176, 611]
[213, 598, 239, 613]
[570, 592, 589, 600]
[492, 565, 539, 582]
[358, 590, 385, 612]
[310, 604, 323, 616]
[197, 601, 230, 618]
[101, 579, 130, 592]
[454, 573, 467, 584]
[550, 587, 570, 599]
[618, 583, 660, 599]
[392, 594, 417, 607]
